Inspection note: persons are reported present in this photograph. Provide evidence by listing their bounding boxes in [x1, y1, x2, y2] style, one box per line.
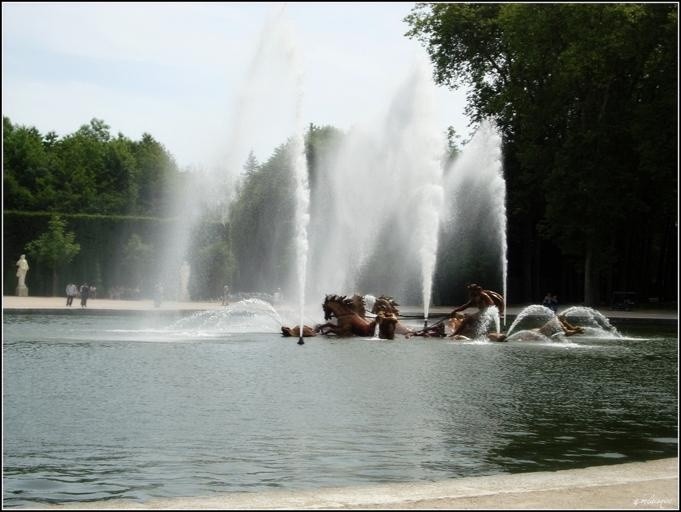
[152, 281, 168, 309]
[15, 254, 30, 288]
[108, 283, 144, 300]
[65, 281, 97, 308]
[447, 283, 495, 339]
[222, 280, 232, 306]
[542, 292, 558, 314]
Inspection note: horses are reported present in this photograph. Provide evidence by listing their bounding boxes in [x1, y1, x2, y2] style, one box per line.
[370, 295, 414, 335]
[313, 294, 375, 337]
[348, 293, 367, 319]
